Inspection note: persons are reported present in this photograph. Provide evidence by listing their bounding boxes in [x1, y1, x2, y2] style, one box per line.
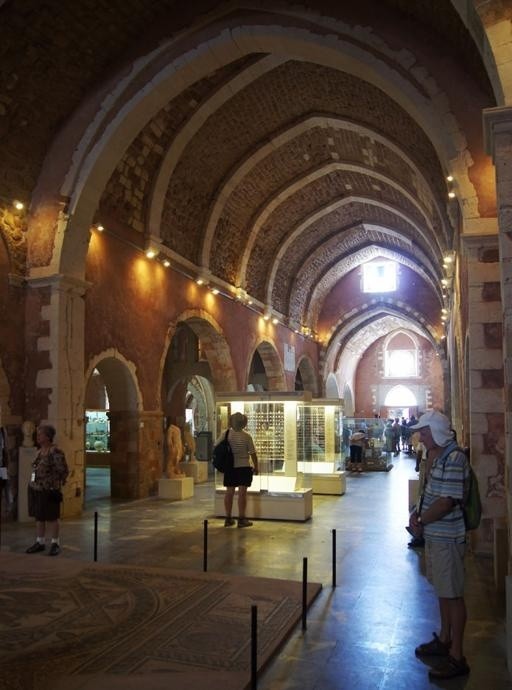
[342, 412, 423, 475]
[25, 424, 68, 556]
[213, 412, 258, 527]
[409, 410, 483, 679]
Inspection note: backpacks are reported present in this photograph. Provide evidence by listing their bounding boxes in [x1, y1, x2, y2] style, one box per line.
[443, 449, 483, 530]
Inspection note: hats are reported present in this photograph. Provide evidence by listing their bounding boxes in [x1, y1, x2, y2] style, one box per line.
[409, 411, 455, 448]
[231, 412, 248, 431]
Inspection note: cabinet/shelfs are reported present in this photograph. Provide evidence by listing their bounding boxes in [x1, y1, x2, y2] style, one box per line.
[342, 417, 394, 472]
[272, 397, 346, 495]
[214, 391, 313, 521]
[85, 409, 110, 465]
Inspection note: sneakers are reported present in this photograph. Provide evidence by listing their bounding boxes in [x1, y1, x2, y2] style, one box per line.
[429, 663, 470, 677]
[415, 637, 449, 657]
[25, 540, 45, 554]
[47, 542, 61, 556]
[238, 518, 253, 527]
[224, 519, 235, 526]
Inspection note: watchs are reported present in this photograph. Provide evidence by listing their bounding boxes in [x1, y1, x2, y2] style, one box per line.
[417, 517, 424, 526]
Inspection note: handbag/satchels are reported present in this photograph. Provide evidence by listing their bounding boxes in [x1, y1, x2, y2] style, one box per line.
[212, 439, 235, 472]
